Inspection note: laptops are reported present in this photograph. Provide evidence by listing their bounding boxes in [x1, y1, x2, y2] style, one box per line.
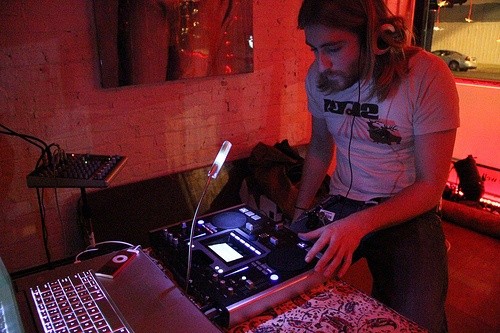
[0, 246, 223, 333]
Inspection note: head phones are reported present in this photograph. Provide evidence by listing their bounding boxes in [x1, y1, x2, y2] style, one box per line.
[360, 0, 396, 54]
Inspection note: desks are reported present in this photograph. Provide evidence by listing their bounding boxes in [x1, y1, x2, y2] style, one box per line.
[141, 246, 434, 333]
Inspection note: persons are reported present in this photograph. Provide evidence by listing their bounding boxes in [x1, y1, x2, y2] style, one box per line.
[289, 0, 461, 333]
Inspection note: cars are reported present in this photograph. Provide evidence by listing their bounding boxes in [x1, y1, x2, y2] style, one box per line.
[432, 49, 477, 73]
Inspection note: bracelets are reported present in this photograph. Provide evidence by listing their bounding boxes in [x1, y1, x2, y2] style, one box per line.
[294, 205, 310, 211]
